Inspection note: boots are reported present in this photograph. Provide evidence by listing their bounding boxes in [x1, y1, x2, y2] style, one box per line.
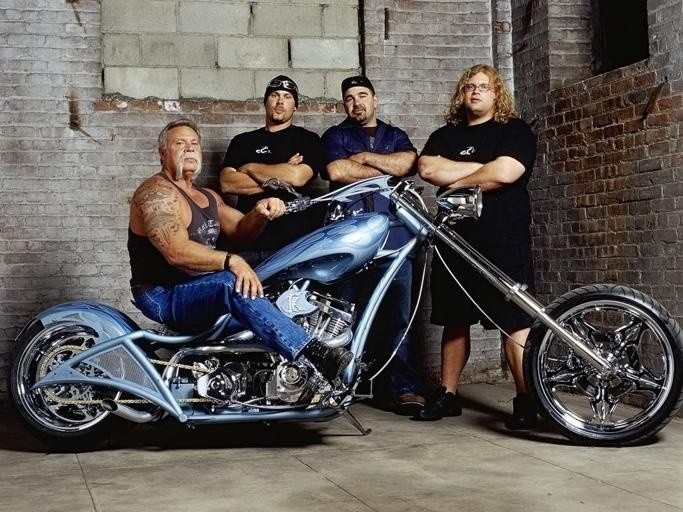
[301, 337, 354, 389]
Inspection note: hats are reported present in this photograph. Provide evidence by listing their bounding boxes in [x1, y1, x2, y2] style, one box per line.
[342, 75, 375, 100]
[263, 75, 298, 109]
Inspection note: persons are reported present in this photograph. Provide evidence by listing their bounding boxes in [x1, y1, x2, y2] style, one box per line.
[127, 118, 353, 377]
[216, 73, 324, 255]
[318, 75, 428, 409]
[415, 61, 555, 430]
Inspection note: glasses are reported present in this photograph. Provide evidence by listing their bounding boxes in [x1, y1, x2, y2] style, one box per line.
[464, 84, 491, 92]
[266, 80, 298, 92]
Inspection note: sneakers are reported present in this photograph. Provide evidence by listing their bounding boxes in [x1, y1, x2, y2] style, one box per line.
[388, 391, 426, 409]
[506, 397, 538, 430]
[414, 385, 462, 421]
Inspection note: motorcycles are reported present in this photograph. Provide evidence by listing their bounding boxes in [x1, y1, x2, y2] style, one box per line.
[6, 173, 682, 448]
